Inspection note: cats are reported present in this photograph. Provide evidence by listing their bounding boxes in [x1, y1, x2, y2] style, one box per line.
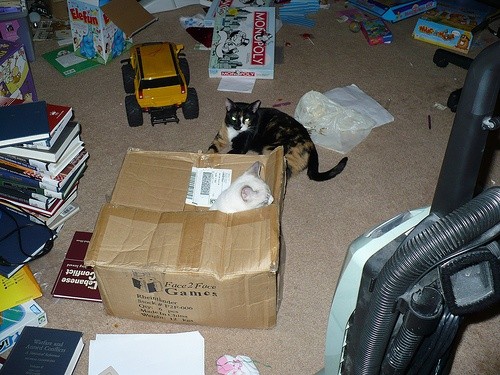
[206, 96, 349, 182]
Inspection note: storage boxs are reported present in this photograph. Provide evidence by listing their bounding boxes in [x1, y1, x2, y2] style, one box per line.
[203, 0, 272, 28]
[84, 144, 288, 331]
[0, 37, 39, 101]
[208, 7, 276, 79]
[344, 0, 437, 25]
[359, 17, 394, 46]
[411, 0, 497, 55]
[67, 0, 159, 65]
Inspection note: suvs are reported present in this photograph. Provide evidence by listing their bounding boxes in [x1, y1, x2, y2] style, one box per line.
[120, 40, 199, 126]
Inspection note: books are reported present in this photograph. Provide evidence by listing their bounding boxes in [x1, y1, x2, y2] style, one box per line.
[1, 100, 89, 375]
[50, 230, 104, 303]
